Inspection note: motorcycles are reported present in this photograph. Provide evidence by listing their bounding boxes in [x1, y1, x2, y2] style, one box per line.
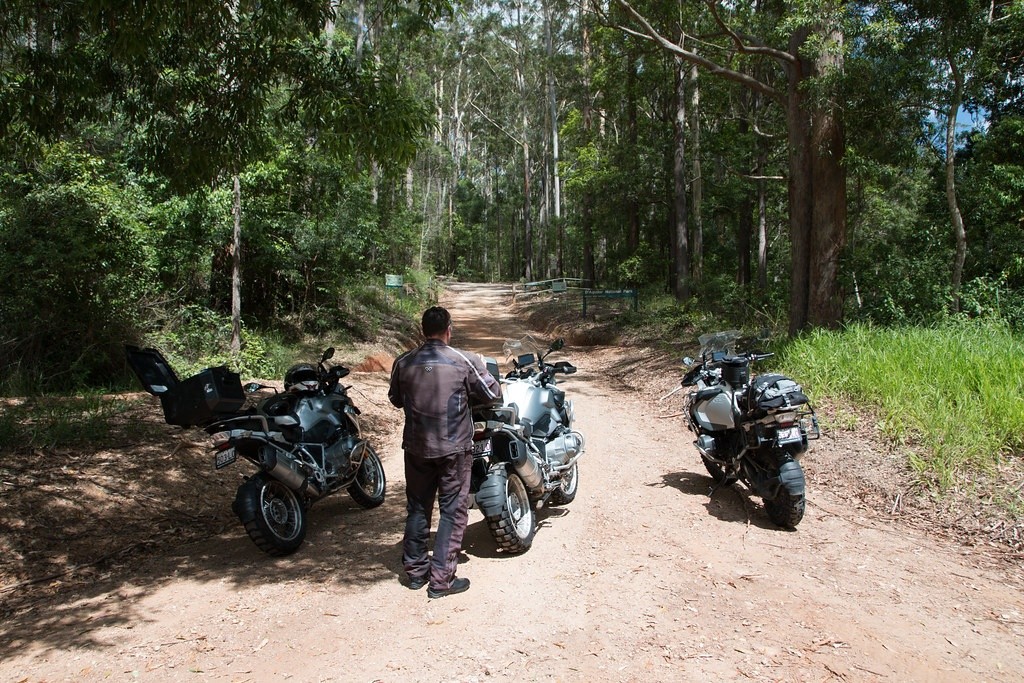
[468, 332, 585, 554]
[132, 347, 386, 557]
[680, 329, 821, 529]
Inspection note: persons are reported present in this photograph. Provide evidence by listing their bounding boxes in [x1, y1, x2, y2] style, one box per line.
[388, 306, 503, 599]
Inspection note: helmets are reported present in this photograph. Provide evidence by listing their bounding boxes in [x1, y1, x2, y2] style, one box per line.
[285, 363, 320, 396]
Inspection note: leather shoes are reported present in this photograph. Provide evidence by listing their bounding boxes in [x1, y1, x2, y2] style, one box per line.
[407, 568, 431, 589]
[428, 578, 470, 598]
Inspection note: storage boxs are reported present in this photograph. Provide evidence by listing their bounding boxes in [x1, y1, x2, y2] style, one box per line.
[121, 342, 246, 425]
